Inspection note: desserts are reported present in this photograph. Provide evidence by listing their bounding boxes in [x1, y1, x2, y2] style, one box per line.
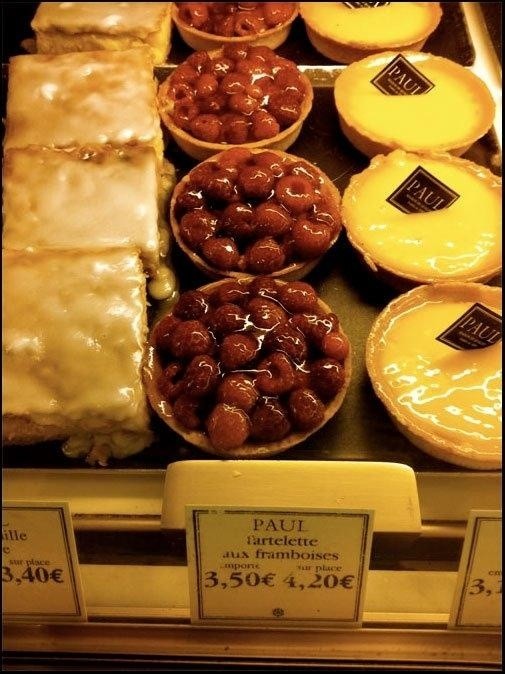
[1, 1, 174, 471]
[153, 1, 349, 449]
